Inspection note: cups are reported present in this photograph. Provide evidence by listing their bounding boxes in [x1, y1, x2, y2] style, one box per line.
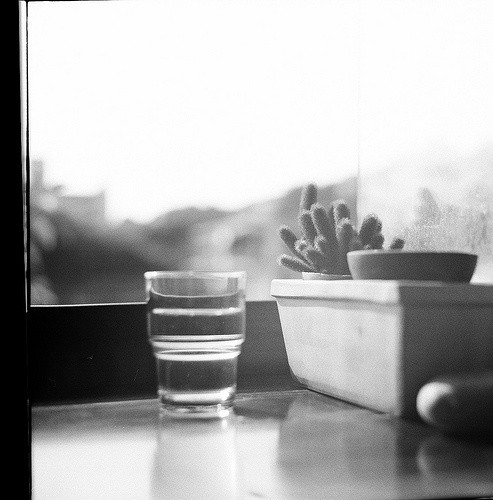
[143, 270, 248, 418]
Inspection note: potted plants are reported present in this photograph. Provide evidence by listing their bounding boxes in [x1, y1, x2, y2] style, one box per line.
[278, 182, 406, 282]
